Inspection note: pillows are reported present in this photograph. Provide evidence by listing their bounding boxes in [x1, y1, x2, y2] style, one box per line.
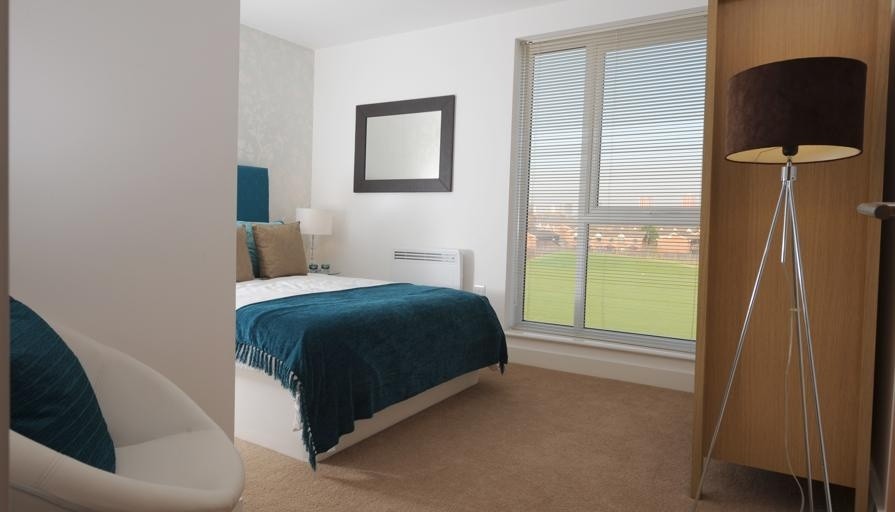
[236, 220, 308, 283]
[10, 296, 116, 474]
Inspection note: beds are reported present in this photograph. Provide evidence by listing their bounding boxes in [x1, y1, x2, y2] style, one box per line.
[235, 165, 508, 473]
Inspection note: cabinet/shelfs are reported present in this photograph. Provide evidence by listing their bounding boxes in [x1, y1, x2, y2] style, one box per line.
[688, 1, 893, 512]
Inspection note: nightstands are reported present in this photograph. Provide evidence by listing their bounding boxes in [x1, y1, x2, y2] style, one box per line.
[308, 269, 341, 276]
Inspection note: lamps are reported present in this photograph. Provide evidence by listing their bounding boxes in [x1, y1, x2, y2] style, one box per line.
[295, 207, 334, 265]
[696, 56, 868, 512]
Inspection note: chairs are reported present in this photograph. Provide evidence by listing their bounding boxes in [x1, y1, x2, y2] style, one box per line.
[9, 320, 246, 512]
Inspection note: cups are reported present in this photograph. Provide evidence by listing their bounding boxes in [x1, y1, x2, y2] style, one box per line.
[310, 264, 329, 274]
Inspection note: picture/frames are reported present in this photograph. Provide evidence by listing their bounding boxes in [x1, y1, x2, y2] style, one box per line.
[353, 95, 456, 194]
